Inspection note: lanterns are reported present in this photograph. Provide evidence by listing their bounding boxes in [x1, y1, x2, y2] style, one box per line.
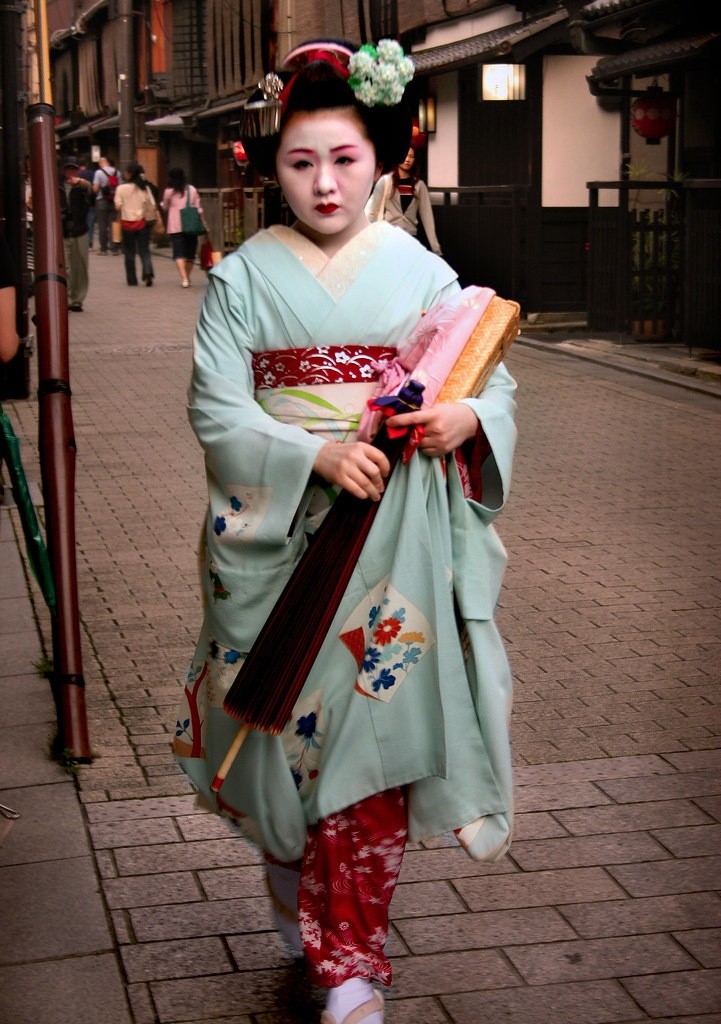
[630, 86, 678, 146]
[232, 139, 250, 164]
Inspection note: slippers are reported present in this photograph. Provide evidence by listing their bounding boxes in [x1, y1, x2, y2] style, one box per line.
[321, 976, 386, 1024]
[263, 862, 306, 950]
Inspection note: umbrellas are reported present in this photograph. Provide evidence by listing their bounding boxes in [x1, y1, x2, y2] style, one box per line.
[211, 379, 427, 796]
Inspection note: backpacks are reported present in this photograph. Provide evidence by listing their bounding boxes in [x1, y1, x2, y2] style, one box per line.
[99, 168, 120, 203]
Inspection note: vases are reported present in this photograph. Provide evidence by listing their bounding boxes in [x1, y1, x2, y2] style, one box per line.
[626, 318, 665, 342]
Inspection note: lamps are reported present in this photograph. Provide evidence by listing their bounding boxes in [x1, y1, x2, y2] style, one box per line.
[418, 87, 437, 134]
[481, 48, 526, 103]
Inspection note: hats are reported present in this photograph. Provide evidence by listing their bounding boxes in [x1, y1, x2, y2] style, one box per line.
[64, 156, 79, 168]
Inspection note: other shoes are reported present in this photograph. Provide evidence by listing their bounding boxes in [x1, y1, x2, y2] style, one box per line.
[181, 280, 192, 287]
[68, 305, 84, 312]
[112, 250, 120, 256]
[96, 250, 107, 256]
[146, 274, 152, 287]
[129, 280, 137, 285]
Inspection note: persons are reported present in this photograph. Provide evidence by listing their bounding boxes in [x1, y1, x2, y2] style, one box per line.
[0, 227, 22, 363]
[371, 140, 445, 255]
[159, 166, 212, 288]
[183, 34, 521, 1024]
[52, 150, 162, 311]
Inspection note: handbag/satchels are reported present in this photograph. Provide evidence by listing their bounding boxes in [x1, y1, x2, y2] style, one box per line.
[200, 231, 213, 270]
[142, 188, 157, 222]
[180, 184, 202, 233]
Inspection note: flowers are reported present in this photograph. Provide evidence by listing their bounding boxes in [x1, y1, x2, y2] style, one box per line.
[624, 162, 688, 315]
[257, 71, 285, 104]
[346, 38, 417, 108]
